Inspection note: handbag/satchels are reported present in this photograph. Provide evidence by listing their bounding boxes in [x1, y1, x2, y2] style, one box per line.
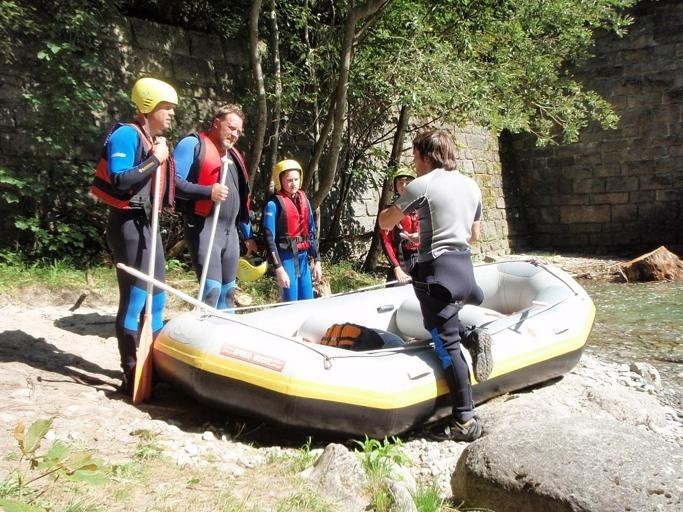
[321, 324, 384, 351]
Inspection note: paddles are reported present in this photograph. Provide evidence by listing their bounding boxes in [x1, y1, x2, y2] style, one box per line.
[133, 135, 165, 404]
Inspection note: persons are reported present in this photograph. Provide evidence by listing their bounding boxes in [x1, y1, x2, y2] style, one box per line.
[95, 79, 180, 395]
[262, 157, 323, 305]
[369, 130, 489, 442]
[167, 103, 260, 311]
[377, 165, 421, 284]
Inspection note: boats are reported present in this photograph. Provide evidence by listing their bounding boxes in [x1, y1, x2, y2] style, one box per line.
[152, 258, 598, 444]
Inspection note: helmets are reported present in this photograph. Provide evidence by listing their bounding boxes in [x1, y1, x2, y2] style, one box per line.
[273, 159, 304, 191]
[393, 169, 416, 187]
[131, 78, 178, 114]
[237, 255, 267, 283]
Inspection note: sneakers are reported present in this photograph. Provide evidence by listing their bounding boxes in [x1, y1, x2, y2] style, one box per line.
[423, 416, 482, 442]
[467, 330, 492, 383]
